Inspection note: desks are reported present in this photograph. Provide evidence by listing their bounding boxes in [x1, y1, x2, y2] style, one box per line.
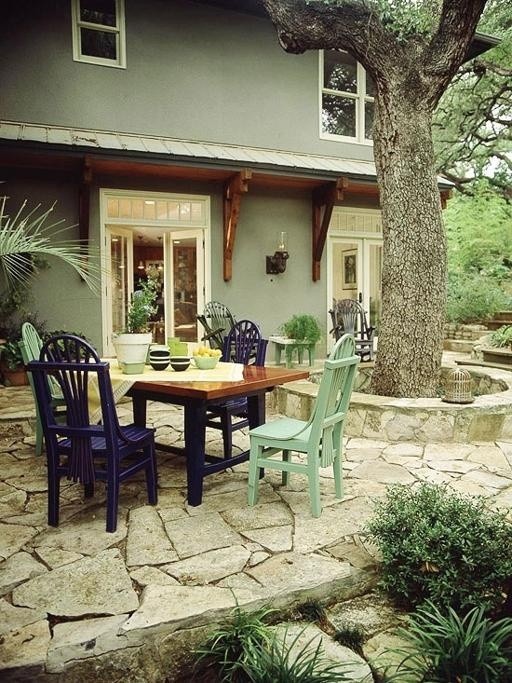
[269, 335, 315, 369]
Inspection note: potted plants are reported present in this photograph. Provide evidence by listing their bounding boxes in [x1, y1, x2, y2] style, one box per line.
[277, 314, 321, 362]
[126, 262, 162, 366]
[0, 341, 29, 386]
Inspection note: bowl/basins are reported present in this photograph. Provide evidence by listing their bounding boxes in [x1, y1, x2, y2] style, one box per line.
[190, 355, 223, 369]
[147, 350, 191, 372]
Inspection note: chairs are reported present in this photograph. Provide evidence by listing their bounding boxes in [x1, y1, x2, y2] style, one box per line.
[196, 301, 259, 365]
[247, 332, 361, 517]
[26, 335, 158, 533]
[328, 298, 376, 361]
[18, 322, 72, 457]
[196, 320, 268, 459]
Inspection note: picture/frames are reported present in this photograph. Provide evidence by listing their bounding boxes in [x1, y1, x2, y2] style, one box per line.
[341, 248, 358, 290]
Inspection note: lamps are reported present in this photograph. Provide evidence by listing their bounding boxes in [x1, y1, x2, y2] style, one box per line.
[265, 230, 290, 275]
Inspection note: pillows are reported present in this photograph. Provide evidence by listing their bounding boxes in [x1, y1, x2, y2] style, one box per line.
[174, 308, 187, 322]
